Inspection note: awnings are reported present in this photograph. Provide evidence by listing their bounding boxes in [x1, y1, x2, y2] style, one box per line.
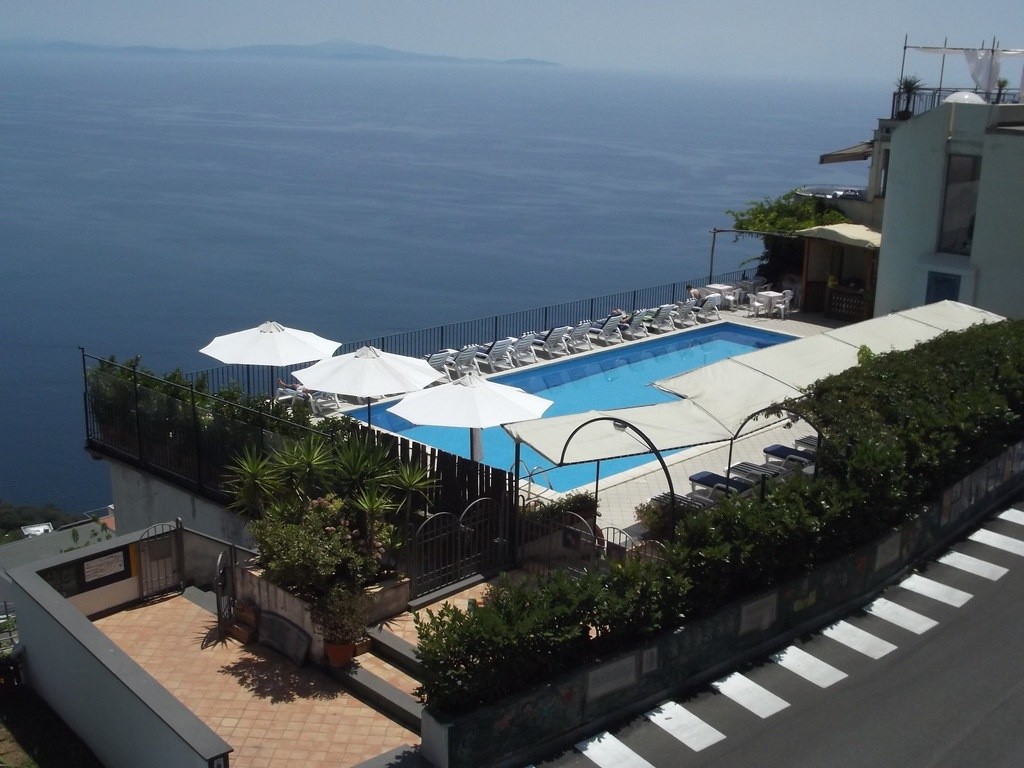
[797, 222, 881, 251]
[500, 300, 1007, 467]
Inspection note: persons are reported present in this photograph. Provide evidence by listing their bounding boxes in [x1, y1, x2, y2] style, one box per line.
[686, 284, 703, 307]
[277, 378, 318, 398]
[611, 309, 639, 331]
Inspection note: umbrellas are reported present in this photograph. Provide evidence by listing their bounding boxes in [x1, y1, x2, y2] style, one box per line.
[291, 345, 445, 428]
[199, 321, 342, 409]
[386, 371, 554, 461]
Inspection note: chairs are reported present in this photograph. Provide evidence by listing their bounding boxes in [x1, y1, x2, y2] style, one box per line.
[701, 274, 805, 322]
[723, 461, 802, 485]
[794, 434, 824, 452]
[688, 470, 768, 503]
[421, 293, 720, 382]
[273, 384, 342, 414]
[763, 443, 817, 469]
[650, 491, 716, 518]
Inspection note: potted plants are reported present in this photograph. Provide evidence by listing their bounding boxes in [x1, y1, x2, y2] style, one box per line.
[519, 491, 601, 544]
[303, 581, 377, 669]
[894, 76, 926, 120]
